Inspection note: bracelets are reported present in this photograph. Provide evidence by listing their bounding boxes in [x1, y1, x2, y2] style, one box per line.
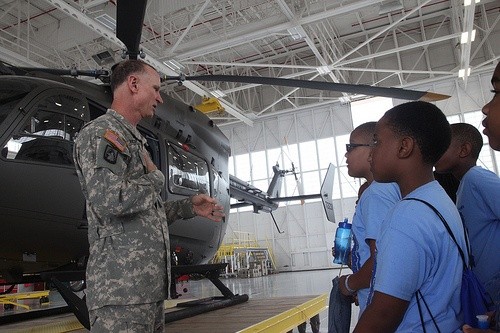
[345, 273, 357, 293]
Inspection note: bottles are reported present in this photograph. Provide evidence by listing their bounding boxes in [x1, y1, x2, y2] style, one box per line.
[331, 218, 352, 264]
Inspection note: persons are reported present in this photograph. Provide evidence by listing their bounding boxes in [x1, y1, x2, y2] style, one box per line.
[72, 60, 225, 333]
[333, 60, 500, 333]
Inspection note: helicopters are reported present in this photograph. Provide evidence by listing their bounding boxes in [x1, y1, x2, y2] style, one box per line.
[0, 0, 452, 328]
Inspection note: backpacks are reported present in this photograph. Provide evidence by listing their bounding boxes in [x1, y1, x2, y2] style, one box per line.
[401, 197, 496, 333]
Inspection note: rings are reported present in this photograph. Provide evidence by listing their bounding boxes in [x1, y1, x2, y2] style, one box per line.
[211, 210, 215, 215]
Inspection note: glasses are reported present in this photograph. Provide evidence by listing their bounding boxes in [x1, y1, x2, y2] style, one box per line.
[345, 143, 369, 152]
